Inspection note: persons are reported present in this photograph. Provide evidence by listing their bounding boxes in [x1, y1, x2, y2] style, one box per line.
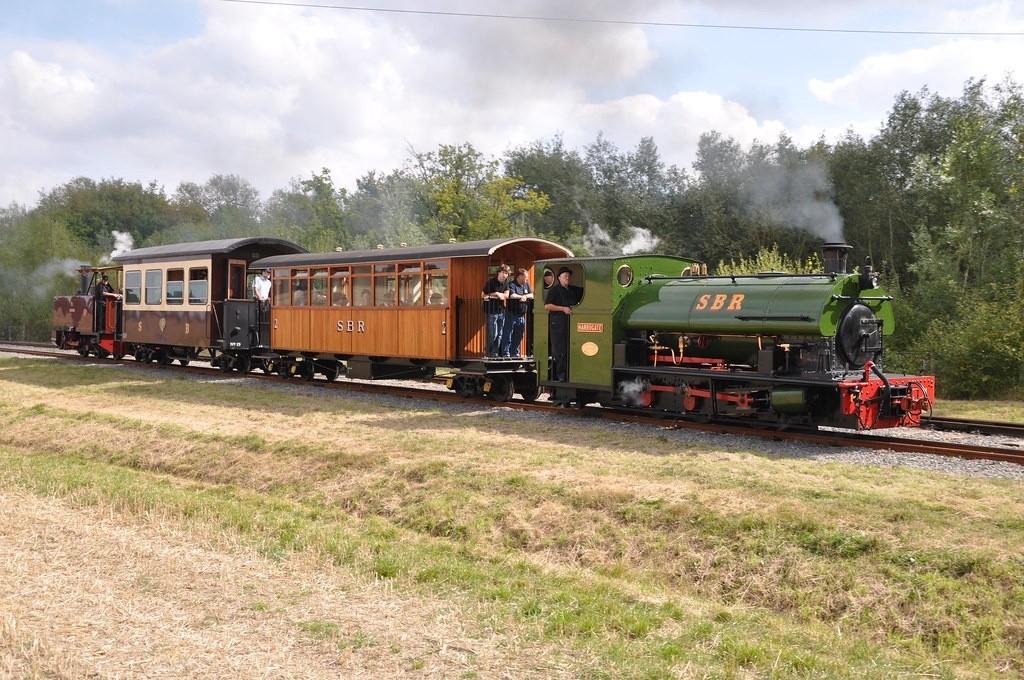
[252, 268, 271, 346]
[96, 275, 119, 332]
[292, 289, 448, 306]
[544, 266, 583, 382]
[481, 264, 535, 357]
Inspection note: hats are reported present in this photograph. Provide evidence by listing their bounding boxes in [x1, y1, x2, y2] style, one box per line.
[103, 275, 108, 280]
[557, 267, 573, 279]
[544, 271, 553, 276]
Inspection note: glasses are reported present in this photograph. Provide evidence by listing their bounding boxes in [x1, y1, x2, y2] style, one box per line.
[264, 270, 271, 273]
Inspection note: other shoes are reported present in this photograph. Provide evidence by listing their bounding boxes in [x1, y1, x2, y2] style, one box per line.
[491, 352, 500, 358]
[511, 353, 519, 357]
[503, 354, 510, 357]
[484, 347, 490, 354]
[558, 377, 566, 382]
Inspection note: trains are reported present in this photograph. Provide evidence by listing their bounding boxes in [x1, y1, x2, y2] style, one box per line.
[49, 236, 937, 432]
